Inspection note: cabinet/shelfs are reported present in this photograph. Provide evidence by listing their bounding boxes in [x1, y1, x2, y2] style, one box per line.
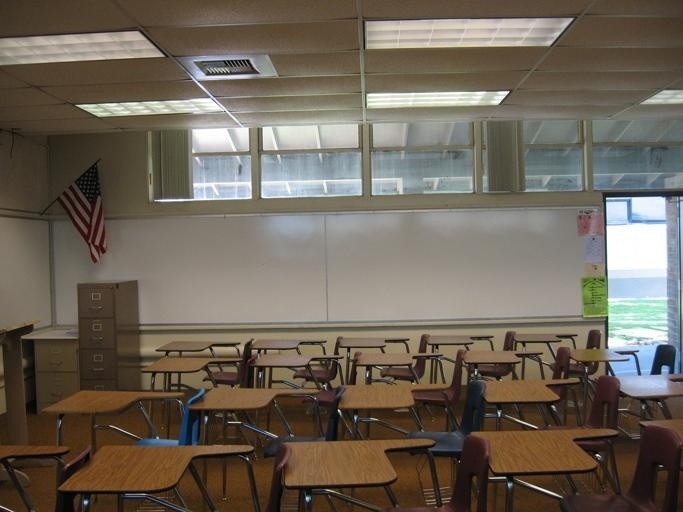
[74, 280, 142, 394]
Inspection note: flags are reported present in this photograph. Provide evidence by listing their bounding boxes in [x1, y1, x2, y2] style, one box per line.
[56, 162, 108, 265]
